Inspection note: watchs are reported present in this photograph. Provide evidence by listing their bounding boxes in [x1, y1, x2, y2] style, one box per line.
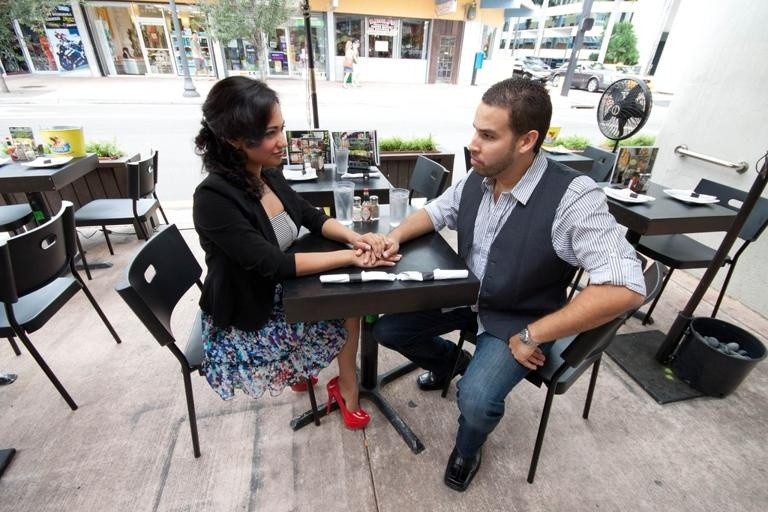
[519, 325, 540, 348]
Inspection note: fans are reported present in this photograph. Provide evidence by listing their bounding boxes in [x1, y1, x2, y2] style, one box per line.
[595, 75, 654, 152]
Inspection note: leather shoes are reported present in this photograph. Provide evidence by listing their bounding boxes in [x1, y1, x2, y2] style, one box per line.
[444, 442, 482, 491]
[418, 350, 472, 390]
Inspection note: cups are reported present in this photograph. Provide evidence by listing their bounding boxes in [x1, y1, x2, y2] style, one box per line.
[389, 187, 409, 228]
[333, 181, 355, 226]
[335, 147, 349, 174]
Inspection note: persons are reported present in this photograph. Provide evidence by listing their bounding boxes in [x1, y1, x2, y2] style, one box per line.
[342, 40, 360, 90]
[190, 32, 215, 82]
[354, 76, 648, 491]
[54, 31, 83, 51]
[121, 48, 134, 59]
[352, 39, 360, 63]
[193, 75, 402, 429]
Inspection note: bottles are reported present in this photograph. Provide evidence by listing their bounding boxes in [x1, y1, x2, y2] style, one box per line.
[306, 149, 324, 171]
[6, 137, 36, 161]
[628, 170, 652, 192]
[353, 197, 362, 222]
[360, 187, 372, 222]
[369, 196, 380, 221]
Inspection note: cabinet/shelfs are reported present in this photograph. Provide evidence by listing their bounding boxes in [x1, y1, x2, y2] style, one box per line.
[169, 29, 218, 78]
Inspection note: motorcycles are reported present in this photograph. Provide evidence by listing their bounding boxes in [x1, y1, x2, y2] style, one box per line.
[56, 39, 87, 69]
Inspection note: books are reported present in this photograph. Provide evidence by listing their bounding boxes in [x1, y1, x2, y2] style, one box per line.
[283, 129, 332, 167]
[609, 145, 658, 188]
[333, 129, 380, 168]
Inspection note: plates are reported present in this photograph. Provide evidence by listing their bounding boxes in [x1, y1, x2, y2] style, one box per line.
[21, 157, 74, 167]
[282, 168, 319, 181]
[662, 188, 720, 204]
[542, 144, 584, 154]
[601, 186, 657, 204]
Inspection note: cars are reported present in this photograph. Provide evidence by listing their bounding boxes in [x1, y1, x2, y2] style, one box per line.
[512, 56, 655, 93]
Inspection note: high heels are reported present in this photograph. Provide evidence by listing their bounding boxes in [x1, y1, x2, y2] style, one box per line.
[272, 369, 318, 392]
[326, 375, 370, 429]
